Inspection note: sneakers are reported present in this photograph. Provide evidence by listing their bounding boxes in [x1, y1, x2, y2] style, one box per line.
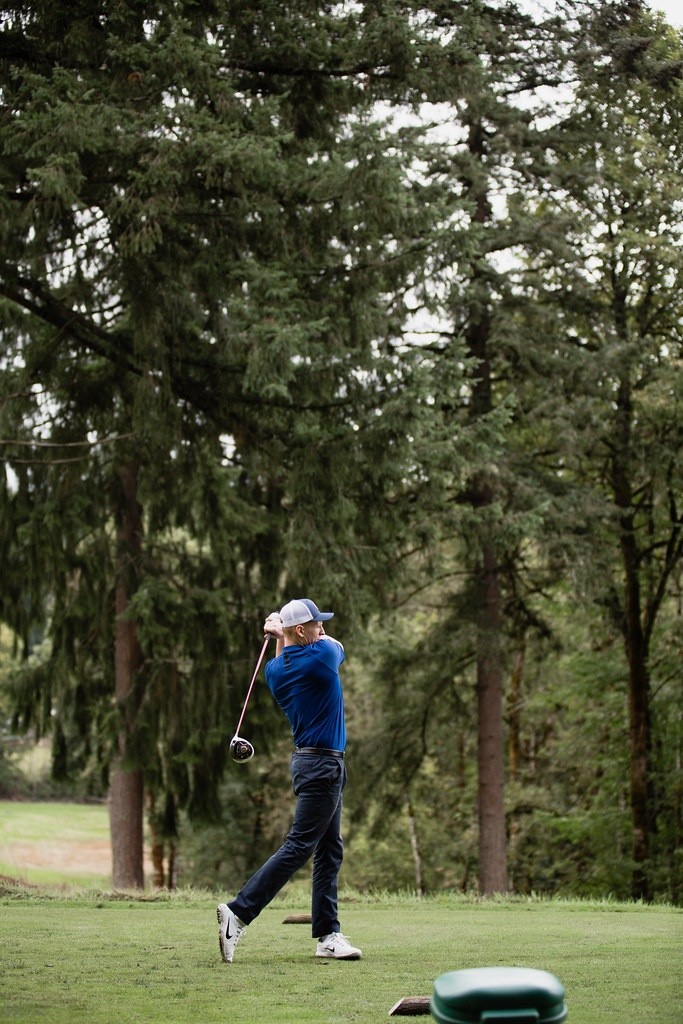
[217, 903, 246, 961]
[315, 932, 362, 959]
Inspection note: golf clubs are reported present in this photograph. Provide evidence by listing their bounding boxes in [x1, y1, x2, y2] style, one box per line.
[228, 608, 275, 766]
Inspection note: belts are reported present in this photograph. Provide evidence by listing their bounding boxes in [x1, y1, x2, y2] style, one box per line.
[295, 747, 346, 758]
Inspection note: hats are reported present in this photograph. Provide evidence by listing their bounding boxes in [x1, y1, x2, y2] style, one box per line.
[280, 599, 335, 627]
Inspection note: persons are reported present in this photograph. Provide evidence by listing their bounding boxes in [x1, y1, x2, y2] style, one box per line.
[217, 598, 362, 963]
